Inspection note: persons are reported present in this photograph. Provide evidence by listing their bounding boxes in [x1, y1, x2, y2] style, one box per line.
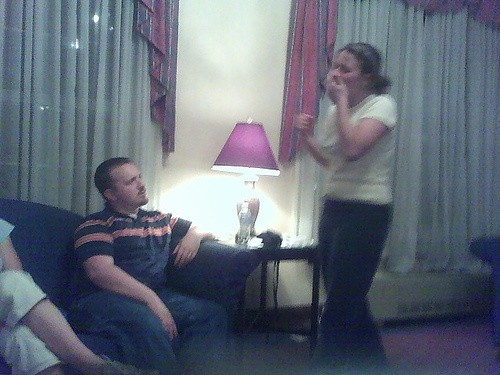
[72, 157, 227, 375]
[0, 219, 142, 375]
[295, 42, 397, 375]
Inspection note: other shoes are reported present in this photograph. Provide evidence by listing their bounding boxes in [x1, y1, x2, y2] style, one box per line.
[99, 354, 160, 375]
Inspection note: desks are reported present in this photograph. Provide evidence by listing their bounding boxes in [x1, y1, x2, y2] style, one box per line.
[225, 232, 321, 360]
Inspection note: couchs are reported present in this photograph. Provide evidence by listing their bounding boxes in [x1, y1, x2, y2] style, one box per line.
[0, 198, 262, 375]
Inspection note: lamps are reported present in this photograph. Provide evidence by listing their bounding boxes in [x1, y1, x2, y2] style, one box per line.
[210, 121, 281, 247]
